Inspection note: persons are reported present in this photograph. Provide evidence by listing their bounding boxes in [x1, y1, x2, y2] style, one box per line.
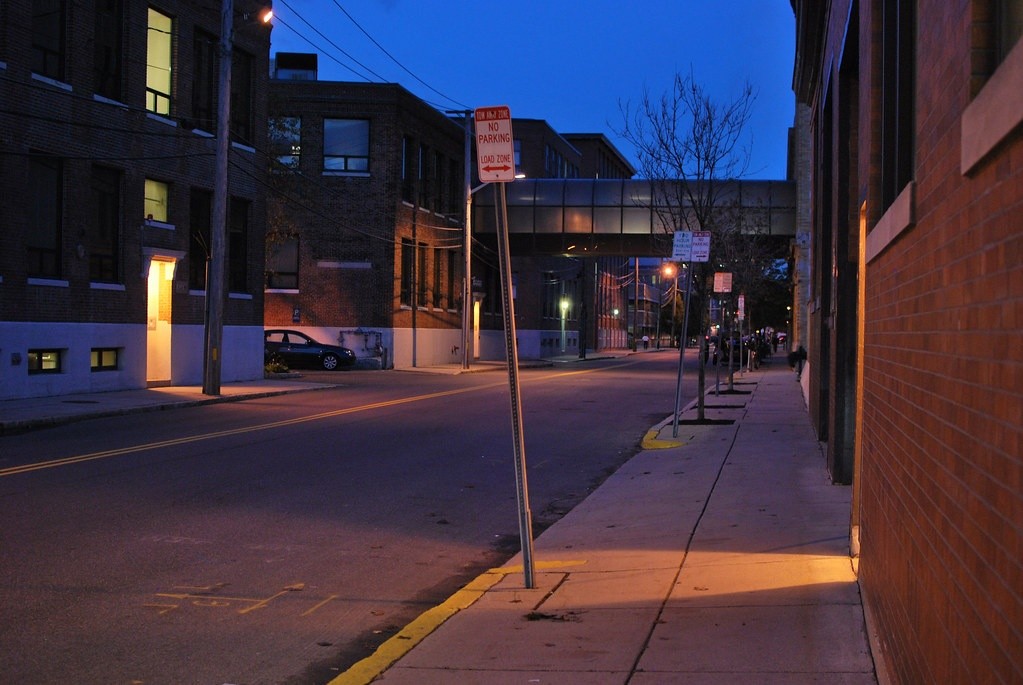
[772, 334, 780, 352]
[675, 336, 681, 350]
[642, 334, 649, 349]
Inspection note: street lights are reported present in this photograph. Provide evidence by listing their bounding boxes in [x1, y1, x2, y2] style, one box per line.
[664, 261, 686, 342]
[203, 0, 274, 396]
[461, 109, 525, 369]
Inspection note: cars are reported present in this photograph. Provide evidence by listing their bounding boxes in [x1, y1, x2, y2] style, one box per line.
[264, 330, 356, 371]
